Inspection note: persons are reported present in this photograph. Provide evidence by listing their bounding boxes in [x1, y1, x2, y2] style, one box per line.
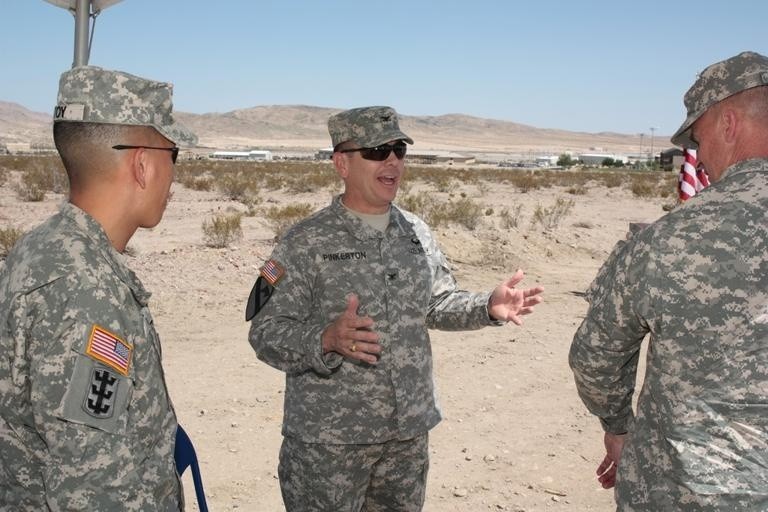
[1, 63, 209, 512]
[568, 49, 768, 512]
[246, 104, 544, 512]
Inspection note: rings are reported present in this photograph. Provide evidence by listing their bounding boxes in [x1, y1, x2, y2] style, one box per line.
[350, 339, 358, 354]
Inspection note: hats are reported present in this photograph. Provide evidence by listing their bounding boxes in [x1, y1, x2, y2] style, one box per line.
[327, 106, 413, 149]
[670, 52, 768, 149]
[53, 65, 198, 148]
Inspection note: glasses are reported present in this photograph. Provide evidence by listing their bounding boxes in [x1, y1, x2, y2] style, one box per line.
[330, 142, 406, 161]
[112, 144, 178, 164]
